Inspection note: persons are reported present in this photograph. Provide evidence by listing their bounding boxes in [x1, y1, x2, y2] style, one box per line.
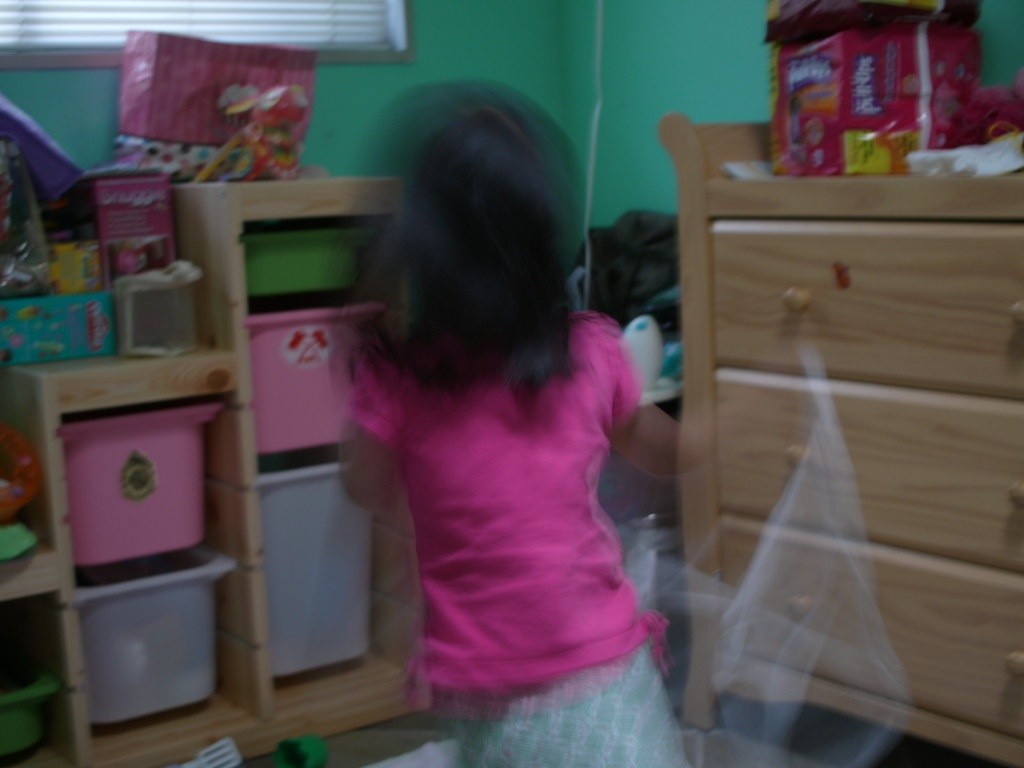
[336, 82, 690, 767]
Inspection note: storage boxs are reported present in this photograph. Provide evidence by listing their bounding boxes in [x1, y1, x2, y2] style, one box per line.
[239, 223, 372, 297]
[1, 669, 65, 760]
[74, 550, 237, 723]
[255, 465, 384, 682]
[244, 305, 388, 455]
[54, 398, 221, 567]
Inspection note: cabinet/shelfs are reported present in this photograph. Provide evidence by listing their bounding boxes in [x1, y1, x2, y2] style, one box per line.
[1, 172, 450, 765]
[656, 110, 1021, 766]
